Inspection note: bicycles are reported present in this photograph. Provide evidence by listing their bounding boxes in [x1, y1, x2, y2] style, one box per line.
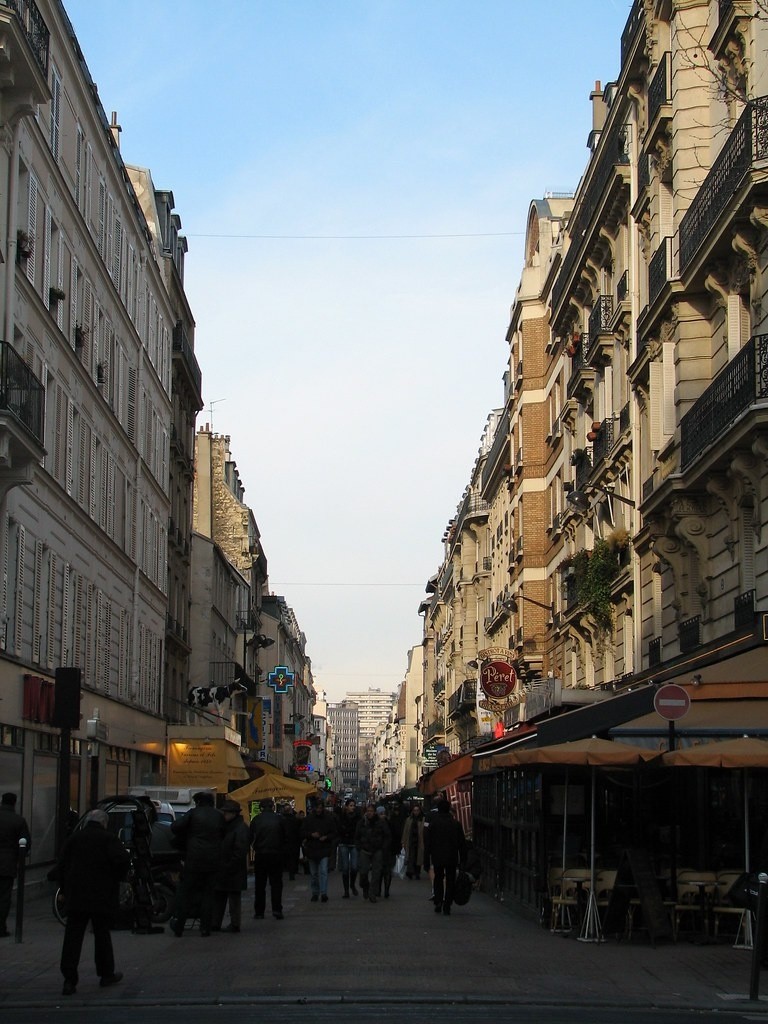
[54, 849, 136, 927]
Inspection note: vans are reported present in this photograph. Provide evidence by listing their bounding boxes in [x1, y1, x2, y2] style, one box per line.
[128, 785, 218, 820]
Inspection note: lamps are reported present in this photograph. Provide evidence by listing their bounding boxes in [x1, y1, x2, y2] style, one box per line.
[437, 698, 449, 706]
[289, 713, 305, 721]
[466, 658, 485, 670]
[325, 755, 332, 761]
[176, 735, 184, 743]
[566, 482, 635, 511]
[306, 732, 315, 737]
[203, 735, 210, 746]
[318, 746, 324, 751]
[690, 674, 704, 688]
[414, 722, 423, 731]
[395, 740, 401, 745]
[501, 596, 555, 618]
[246, 634, 275, 655]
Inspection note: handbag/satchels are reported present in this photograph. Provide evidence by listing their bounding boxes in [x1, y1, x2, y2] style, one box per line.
[455, 871, 472, 905]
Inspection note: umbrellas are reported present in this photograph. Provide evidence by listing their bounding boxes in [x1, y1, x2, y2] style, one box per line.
[660, 734, 768, 947]
[490, 736, 665, 943]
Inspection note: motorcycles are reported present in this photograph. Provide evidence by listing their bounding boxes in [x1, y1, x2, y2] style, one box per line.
[133, 852, 173, 923]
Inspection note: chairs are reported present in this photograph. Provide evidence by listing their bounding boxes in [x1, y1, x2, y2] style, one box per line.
[547, 866, 753, 950]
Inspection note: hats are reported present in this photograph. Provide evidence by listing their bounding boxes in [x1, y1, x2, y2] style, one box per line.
[376, 808, 385, 815]
[221, 800, 242, 812]
[2, 793, 17, 804]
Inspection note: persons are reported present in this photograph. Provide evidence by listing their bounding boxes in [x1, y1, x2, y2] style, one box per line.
[277, 793, 472, 916]
[213, 800, 251, 935]
[0, 793, 32, 937]
[251, 800, 291, 921]
[170, 791, 226, 938]
[57, 810, 128, 997]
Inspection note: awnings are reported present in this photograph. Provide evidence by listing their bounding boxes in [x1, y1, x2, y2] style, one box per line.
[413, 715, 540, 798]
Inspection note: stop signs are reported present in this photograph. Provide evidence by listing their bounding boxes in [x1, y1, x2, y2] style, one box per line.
[654, 685, 690, 720]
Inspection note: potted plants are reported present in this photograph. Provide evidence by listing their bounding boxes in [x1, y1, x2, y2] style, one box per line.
[557, 558, 575, 581]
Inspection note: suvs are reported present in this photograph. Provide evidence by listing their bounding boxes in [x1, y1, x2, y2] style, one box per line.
[107, 801, 177, 845]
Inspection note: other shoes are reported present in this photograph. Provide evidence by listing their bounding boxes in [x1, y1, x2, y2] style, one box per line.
[223, 924, 240, 933]
[311, 894, 318, 901]
[351, 886, 358, 896]
[63, 986, 77, 995]
[436, 905, 442, 914]
[342, 893, 351, 898]
[170, 919, 183, 932]
[101, 972, 122, 985]
[443, 905, 451, 915]
[255, 914, 264, 919]
[321, 894, 330, 903]
[368, 891, 376, 901]
[364, 893, 368, 901]
[202, 931, 210, 936]
[0, 931, 10, 937]
[274, 911, 284, 920]
